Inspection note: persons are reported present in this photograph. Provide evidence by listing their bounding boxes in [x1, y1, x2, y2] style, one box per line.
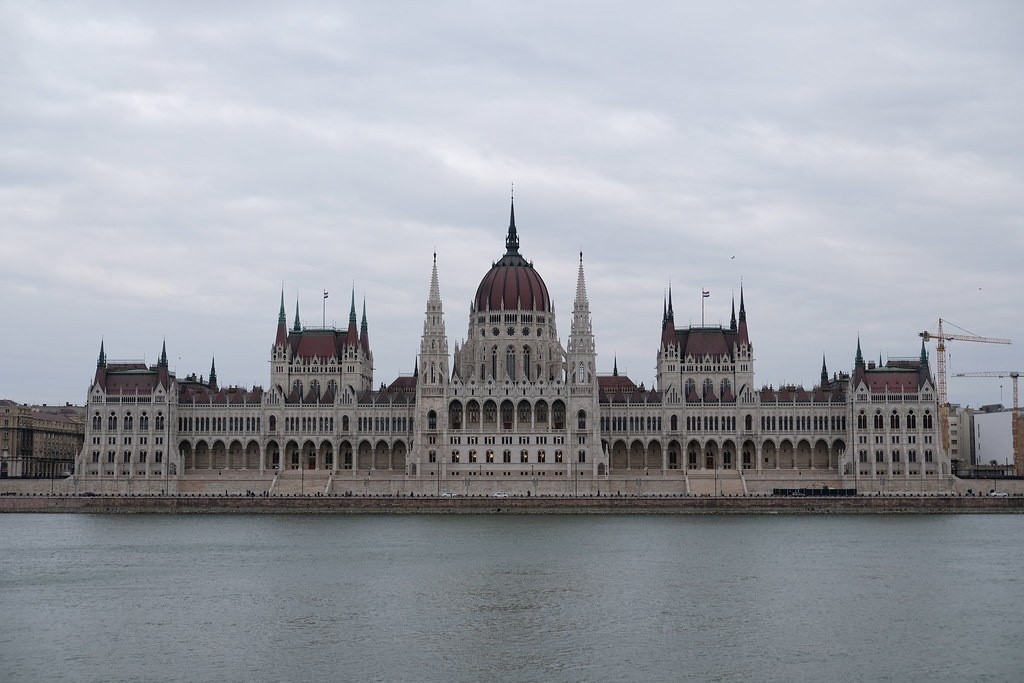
[979, 490, 982, 497]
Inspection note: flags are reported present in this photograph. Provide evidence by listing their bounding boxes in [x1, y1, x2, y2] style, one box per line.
[324, 292, 328, 298]
[703, 291, 709, 297]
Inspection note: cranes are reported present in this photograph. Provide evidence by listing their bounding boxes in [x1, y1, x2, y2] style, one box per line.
[951, 371, 1024, 476]
[918, 317, 1013, 457]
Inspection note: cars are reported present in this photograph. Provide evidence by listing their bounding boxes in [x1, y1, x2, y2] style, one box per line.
[990, 491, 1008, 497]
[442, 492, 457, 498]
[492, 492, 508, 498]
[791, 492, 805, 497]
